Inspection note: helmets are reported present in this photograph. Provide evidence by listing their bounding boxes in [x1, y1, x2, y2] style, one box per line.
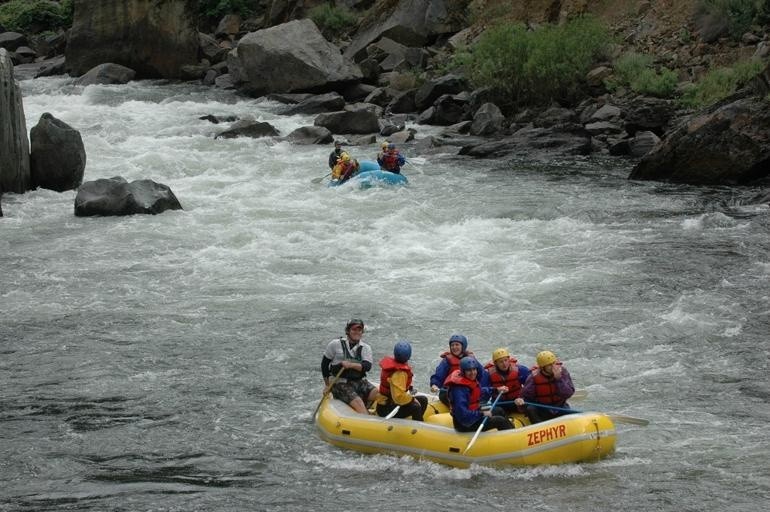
[394, 342, 411, 362]
[345, 318, 365, 335]
[460, 356, 478, 376]
[381, 142, 395, 149]
[449, 335, 467, 353]
[536, 351, 557, 367]
[340, 152, 350, 161]
[492, 348, 510, 364]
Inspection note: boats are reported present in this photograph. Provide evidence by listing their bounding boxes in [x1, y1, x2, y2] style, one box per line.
[329, 160, 407, 190]
[316, 383, 618, 468]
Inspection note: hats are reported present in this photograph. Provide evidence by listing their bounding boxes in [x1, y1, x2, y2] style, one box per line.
[335, 140, 342, 147]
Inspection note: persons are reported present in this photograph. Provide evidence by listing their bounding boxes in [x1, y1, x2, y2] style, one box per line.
[443, 355, 516, 432]
[329, 139, 347, 169]
[379, 142, 400, 159]
[513, 351, 576, 424]
[484, 348, 543, 429]
[377, 342, 428, 421]
[331, 152, 359, 185]
[430, 334, 485, 410]
[321, 318, 378, 414]
[377, 143, 405, 173]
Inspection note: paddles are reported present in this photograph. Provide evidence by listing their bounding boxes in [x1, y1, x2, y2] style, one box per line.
[463, 388, 505, 455]
[312, 172, 335, 184]
[405, 159, 424, 174]
[382, 390, 413, 421]
[517, 402, 651, 427]
